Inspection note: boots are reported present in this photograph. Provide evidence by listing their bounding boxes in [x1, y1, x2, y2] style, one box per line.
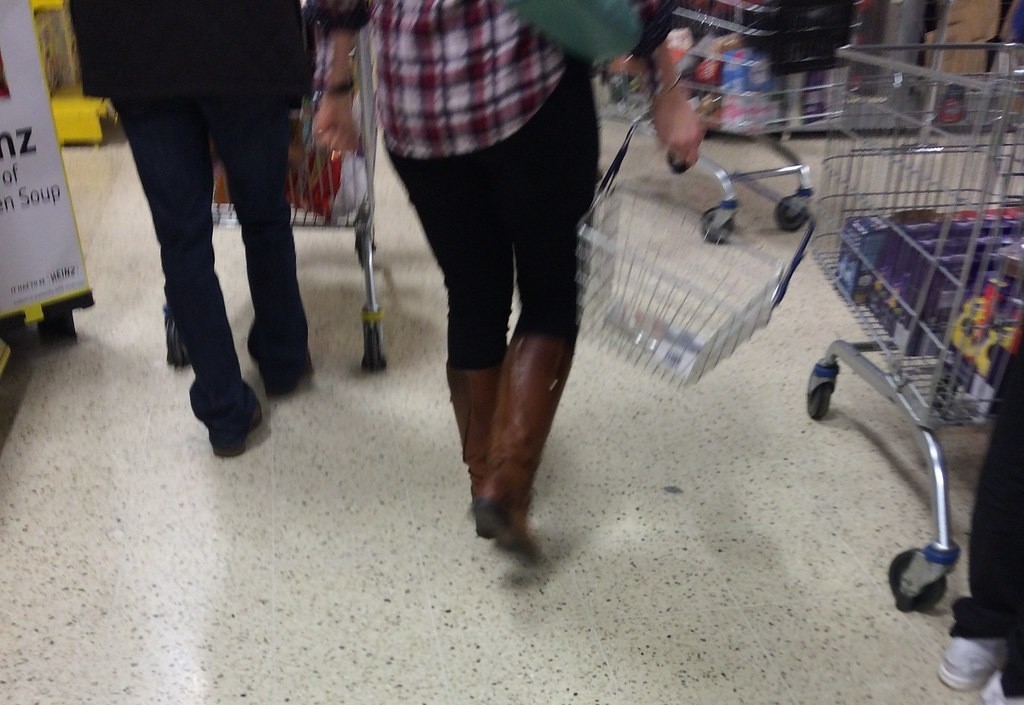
[449, 360, 500, 499]
[474, 331, 573, 564]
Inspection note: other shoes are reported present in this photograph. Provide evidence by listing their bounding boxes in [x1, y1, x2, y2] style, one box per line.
[213, 398, 262, 456]
[264, 349, 313, 396]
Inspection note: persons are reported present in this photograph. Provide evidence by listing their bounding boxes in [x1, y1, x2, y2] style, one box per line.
[65, 0, 371, 458]
[371, 0, 703, 558]
[938, 312, 1024, 705]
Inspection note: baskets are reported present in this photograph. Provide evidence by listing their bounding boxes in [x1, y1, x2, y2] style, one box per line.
[575, 119, 817, 392]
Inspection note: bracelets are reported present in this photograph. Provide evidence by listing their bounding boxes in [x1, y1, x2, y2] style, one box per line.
[656, 70, 684, 101]
[321, 73, 354, 97]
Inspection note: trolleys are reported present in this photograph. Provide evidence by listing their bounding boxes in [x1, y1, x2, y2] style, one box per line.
[805, 40, 1024, 613]
[164, 12, 387, 371]
[597, 0, 874, 245]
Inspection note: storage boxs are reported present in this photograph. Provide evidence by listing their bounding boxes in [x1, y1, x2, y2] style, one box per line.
[666, 37, 777, 133]
[831, 205, 1024, 421]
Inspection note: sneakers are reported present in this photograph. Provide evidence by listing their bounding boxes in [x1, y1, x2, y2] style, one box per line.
[939, 637, 1024, 705]
